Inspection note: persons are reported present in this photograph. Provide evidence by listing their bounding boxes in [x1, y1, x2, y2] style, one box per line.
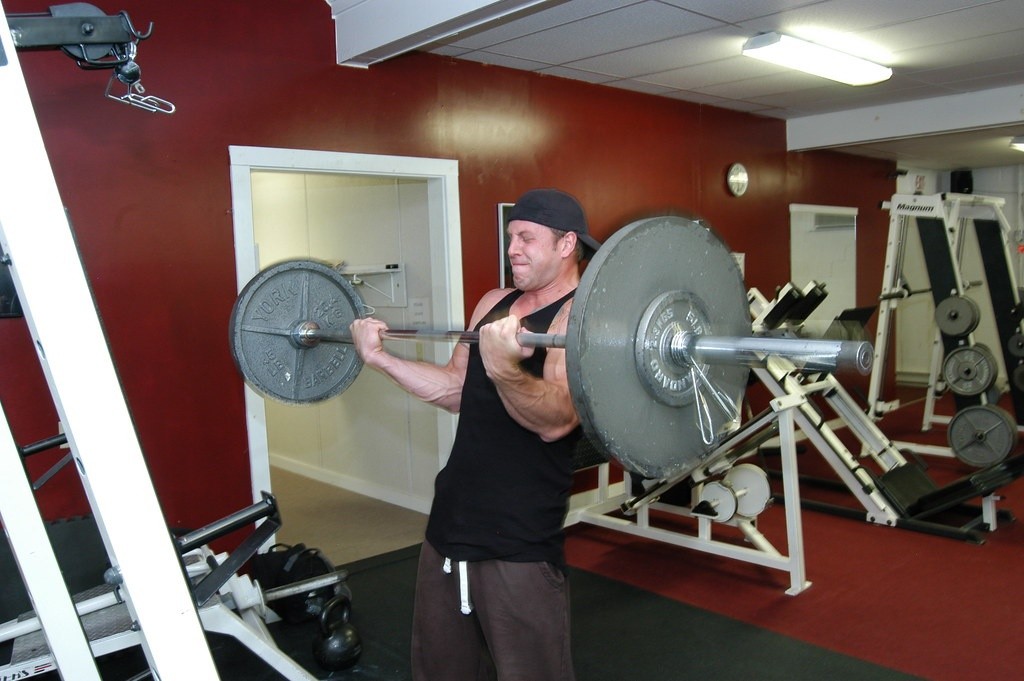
[348, 189, 601, 681]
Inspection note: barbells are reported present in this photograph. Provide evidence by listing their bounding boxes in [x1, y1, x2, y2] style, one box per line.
[228, 215, 877, 480]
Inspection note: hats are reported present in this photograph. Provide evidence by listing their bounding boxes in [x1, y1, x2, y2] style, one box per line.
[507, 188, 602, 262]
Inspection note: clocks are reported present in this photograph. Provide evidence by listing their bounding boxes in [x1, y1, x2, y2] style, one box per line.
[726, 162, 750, 198]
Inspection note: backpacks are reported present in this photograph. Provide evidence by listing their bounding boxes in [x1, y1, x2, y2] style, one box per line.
[249, 543, 351, 626]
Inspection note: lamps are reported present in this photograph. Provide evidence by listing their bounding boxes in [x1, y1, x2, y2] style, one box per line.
[741, 31, 893, 88]
[1007, 136, 1024, 152]
